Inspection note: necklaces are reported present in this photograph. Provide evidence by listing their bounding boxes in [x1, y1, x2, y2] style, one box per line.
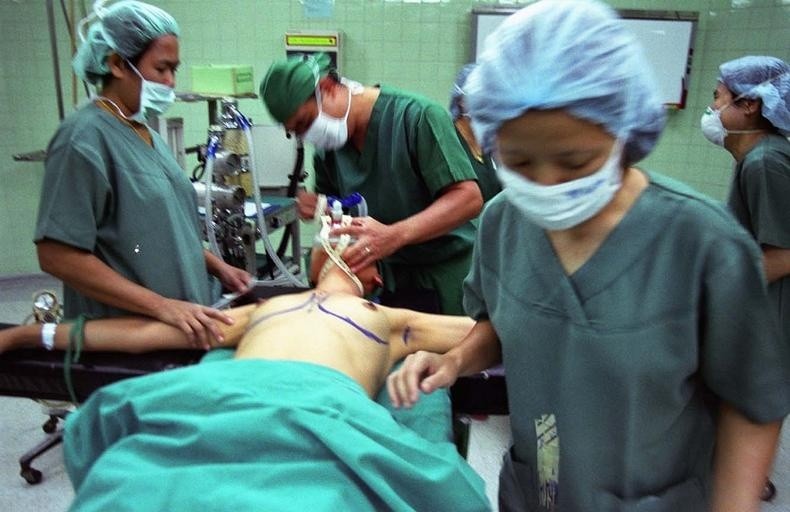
[458, 123, 483, 164]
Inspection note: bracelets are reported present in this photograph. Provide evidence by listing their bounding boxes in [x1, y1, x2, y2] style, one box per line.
[41, 319, 58, 354]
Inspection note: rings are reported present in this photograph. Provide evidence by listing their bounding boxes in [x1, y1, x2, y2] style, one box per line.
[365, 246, 373, 255]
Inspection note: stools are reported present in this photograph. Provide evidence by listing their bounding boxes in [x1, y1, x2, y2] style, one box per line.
[17, 406, 75, 484]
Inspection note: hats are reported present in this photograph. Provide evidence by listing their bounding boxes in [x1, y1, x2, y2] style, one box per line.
[717, 55, 790, 136]
[72, 1, 180, 84]
[259, 53, 332, 122]
[464, 0, 667, 166]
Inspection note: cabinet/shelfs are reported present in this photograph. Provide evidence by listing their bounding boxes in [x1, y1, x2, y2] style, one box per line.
[195, 191, 304, 293]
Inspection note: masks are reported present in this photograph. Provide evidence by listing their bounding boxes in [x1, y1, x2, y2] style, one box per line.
[496, 135, 627, 232]
[119, 61, 175, 124]
[701, 88, 755, 148]
[303, 90, 351, 151]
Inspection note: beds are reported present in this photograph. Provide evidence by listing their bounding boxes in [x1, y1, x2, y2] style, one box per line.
[0, 323, 512, 511]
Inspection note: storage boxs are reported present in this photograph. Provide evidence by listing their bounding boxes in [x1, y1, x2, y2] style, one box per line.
[188, 63, 256, 100]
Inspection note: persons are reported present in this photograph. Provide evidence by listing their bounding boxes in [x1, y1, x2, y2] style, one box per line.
[258, 45, 486, 315]
[31, 1, 252, 353]
[0, 228, 480, 406]
[698, 53, 790, 346]
[384, 0, 789, 511]
[444, 58, 505, 204]
